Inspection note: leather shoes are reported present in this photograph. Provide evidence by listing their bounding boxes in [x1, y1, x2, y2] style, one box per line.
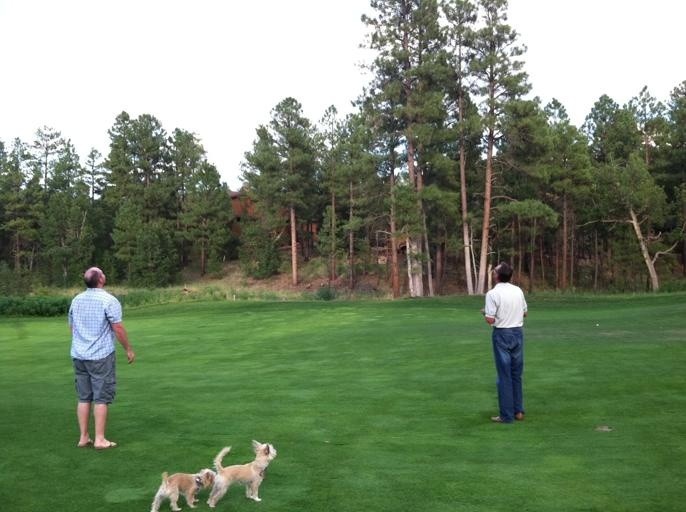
[491, 412, 524, 422]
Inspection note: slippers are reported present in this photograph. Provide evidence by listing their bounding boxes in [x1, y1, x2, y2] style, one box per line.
[96, 441, 116, 450]
[78, 440, 93, 448]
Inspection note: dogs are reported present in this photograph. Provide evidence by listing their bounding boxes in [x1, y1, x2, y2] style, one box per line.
[148, 467, 215, 512]
[206, 438, 278, 510]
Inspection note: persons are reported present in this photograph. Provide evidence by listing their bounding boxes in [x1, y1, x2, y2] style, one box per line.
[480, 261, 528, 423]
[66, 265, 135, 451]
